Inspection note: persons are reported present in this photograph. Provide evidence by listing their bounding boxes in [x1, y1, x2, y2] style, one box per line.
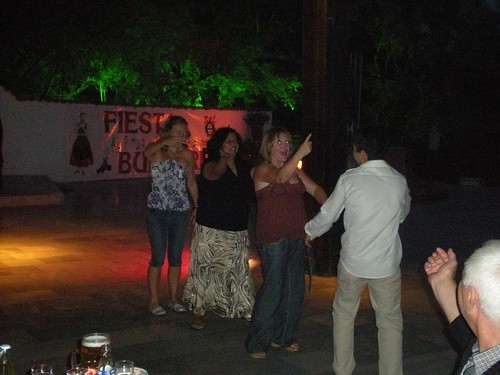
[304, 127, 412, 375]
[182, 127, 257, 328]
[144, 115, 197, 316]
[245, 128, 329, 358]
[423, 239, 500, 375]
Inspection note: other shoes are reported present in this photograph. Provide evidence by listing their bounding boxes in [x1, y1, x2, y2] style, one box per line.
[271, 340, 302, 352]
[249, 351, 266, 358]
[147, 303, 167, 315]
[167, 300, 186, 313]
[190, 313, 205, 330]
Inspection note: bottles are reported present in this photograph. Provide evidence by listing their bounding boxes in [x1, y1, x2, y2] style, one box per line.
[97, 345, 115, 375]
[0, 344, 16, 375]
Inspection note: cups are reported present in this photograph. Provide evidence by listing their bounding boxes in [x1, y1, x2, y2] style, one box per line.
[80, 332, 112, 370]
[115, 360, 135, 375]
[65, 366, 89, 375]
[29, 363, 54, 375]
